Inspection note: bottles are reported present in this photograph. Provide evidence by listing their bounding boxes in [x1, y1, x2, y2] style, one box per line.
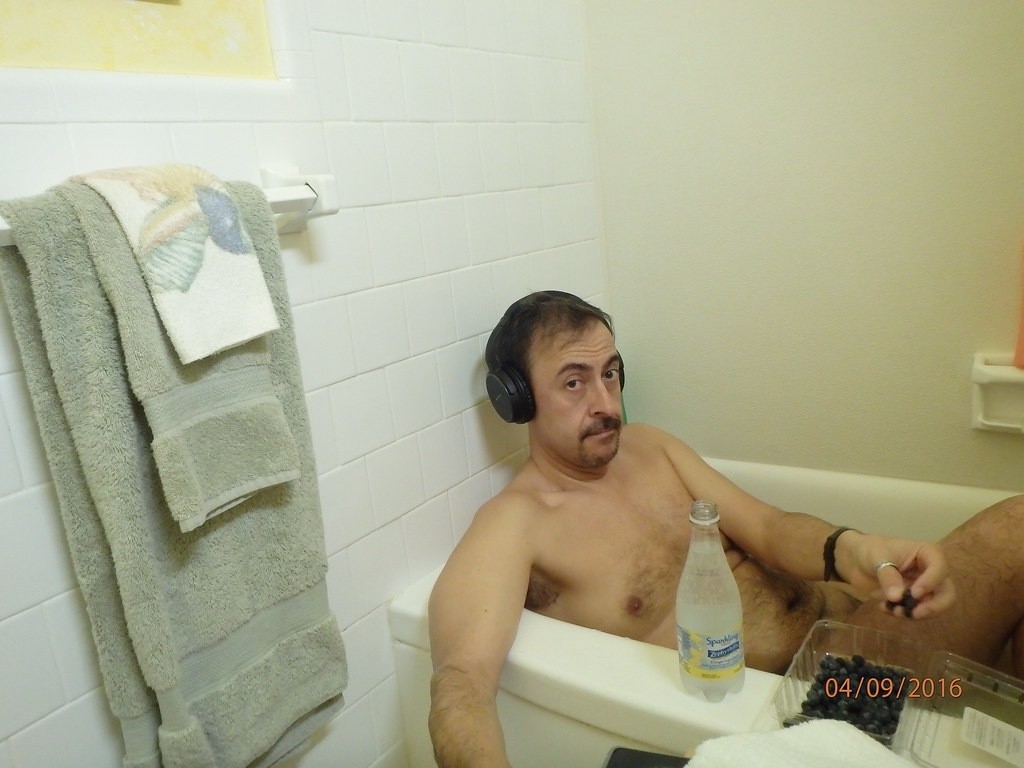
[675, 501, 745, 702]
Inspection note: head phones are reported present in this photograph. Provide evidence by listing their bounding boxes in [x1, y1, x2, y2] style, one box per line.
[485, 290, 625, 425]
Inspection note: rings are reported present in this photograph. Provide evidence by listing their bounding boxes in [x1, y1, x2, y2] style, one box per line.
[876, 561, 899, 573]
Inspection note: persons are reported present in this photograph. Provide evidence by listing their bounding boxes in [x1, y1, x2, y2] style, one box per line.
[427, 290, 1024, 768]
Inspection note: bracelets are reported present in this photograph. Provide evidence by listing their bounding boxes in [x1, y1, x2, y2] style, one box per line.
[824, 528, 849, 582]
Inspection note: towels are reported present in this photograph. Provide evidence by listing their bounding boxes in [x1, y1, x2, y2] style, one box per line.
[682, 718, 917, 768]
[0, 161, 353, 768]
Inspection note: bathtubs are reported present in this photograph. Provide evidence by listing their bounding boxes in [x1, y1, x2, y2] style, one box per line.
[382, 449, 1024, 768]
